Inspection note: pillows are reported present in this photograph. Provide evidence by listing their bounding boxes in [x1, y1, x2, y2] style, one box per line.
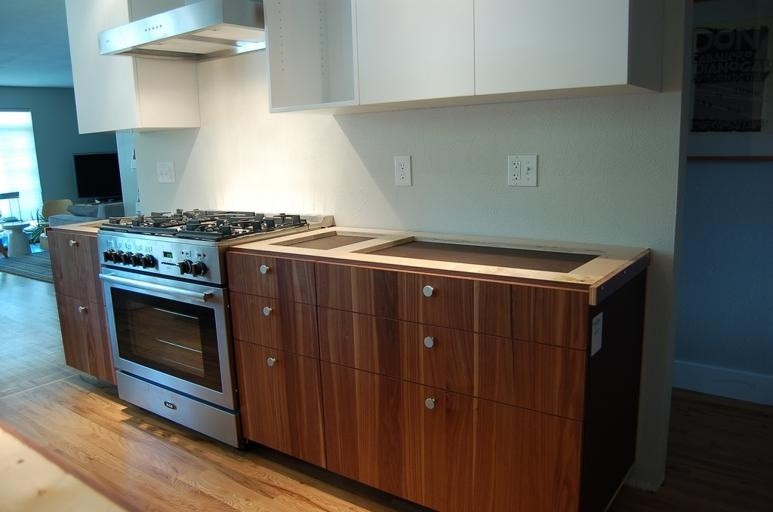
[66, 203, 98, 216]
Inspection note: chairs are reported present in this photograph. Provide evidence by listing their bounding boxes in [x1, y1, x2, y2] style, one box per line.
[39, 198, 74, 250]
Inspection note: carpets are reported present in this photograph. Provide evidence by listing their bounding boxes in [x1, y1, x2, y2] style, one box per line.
[0, 250, 55, 284]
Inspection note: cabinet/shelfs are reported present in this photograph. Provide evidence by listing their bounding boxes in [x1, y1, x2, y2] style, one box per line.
[45, 226, 117, 387]
[63, 0, 204, 137]
[224, 243, 327, 470]
[261, 0, 360, 115]
[355, 0, 665, 110]
[314, 255, 648, 512]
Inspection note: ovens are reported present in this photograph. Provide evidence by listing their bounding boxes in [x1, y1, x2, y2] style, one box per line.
[99, 268, 249, 414]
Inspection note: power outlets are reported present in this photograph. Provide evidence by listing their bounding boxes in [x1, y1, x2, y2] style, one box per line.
[507, 154, 538, 188]
[394, 156, 412, 187]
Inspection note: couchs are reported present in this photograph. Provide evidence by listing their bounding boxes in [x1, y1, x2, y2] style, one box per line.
[47, 201, 124, 227]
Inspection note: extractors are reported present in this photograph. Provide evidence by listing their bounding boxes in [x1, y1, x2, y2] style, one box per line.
[98, 0, 267, 62]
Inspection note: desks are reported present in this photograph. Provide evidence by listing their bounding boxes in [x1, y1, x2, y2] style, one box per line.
[1, 221, 32, 257]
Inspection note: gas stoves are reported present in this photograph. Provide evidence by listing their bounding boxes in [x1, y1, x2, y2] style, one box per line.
[98, 209, 336, 244]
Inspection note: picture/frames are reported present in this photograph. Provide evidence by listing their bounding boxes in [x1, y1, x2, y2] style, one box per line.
[684, 18, 773, 159]
